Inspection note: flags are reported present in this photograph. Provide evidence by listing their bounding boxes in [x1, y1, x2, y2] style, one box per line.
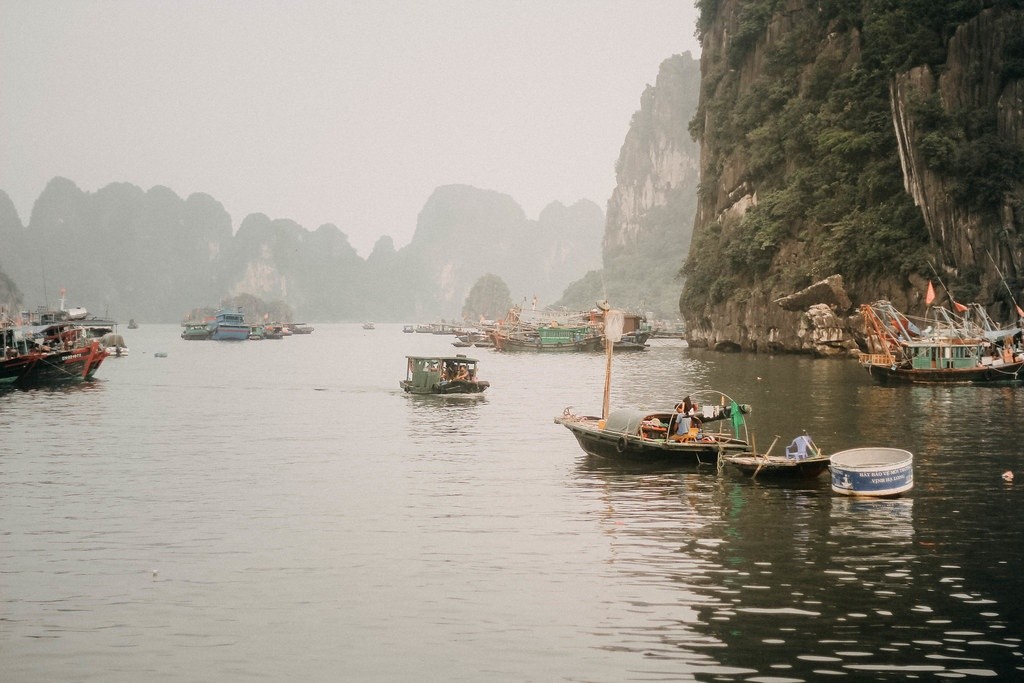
[926, 281, 935, 304]
[954, 302, 967, 311]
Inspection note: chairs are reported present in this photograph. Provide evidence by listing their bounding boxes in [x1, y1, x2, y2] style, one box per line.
[682, 428, 698, 443]
[786, 435, 812, 460]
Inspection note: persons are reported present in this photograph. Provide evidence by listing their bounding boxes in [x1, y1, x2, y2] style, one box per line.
[444, 361, 457, 380]
[669, 403, 691, 441]
[456, 365, 470, 381]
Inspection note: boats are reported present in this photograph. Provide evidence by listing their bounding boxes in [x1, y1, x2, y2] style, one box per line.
[181, 295, 315, 342]
[362, 321, 376, 329]
[724, 429, 831, 481]
[0, 249, 130, 396]
[553, 308, 753, 468]
[850, 248, 1024, 389]
[399, 353, 491, 397]
[402, 294, 661, 356]
